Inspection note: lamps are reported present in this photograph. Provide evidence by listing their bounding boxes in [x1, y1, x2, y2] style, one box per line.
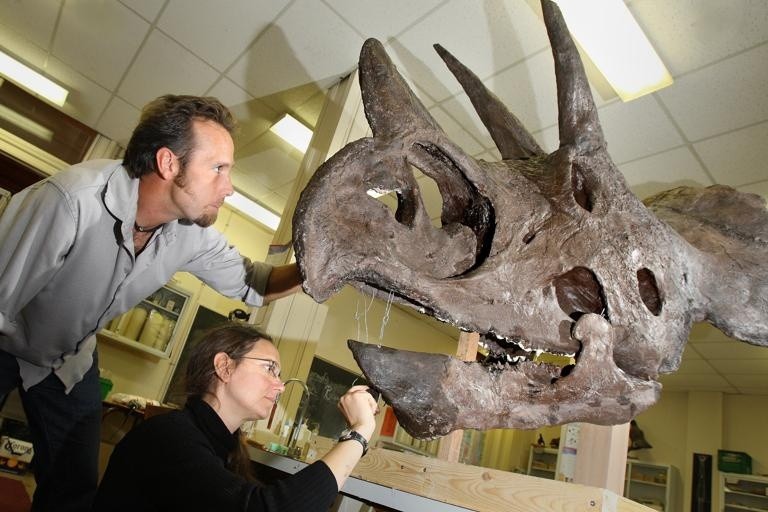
[267, 111, 388, 200]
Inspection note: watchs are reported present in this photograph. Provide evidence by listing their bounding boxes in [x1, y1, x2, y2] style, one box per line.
[333, 428, 370, 460]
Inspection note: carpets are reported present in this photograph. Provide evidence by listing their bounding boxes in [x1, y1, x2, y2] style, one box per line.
[0, 476, 33, 510]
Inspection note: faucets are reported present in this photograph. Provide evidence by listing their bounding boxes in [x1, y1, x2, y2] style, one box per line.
[267, 378, 310, 448]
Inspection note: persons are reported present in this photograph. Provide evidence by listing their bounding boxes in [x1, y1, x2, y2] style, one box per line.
[88, 323, 382, 512]
[0, 92, 306, 511]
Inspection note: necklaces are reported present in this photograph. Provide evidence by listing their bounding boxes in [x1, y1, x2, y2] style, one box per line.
[133, 220, 156, 232]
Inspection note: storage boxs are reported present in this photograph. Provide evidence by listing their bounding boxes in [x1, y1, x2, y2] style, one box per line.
[715, 449, 752, 474]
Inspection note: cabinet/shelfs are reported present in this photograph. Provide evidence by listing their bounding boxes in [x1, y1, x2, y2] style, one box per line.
[525, 442, 558, 480]
[718, 472, 768, 512]
[96, 282, 195, 365]
[624, 457, 681, 512]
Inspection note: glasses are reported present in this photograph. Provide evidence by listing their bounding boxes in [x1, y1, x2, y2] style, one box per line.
[239, 356, 280, 377]
